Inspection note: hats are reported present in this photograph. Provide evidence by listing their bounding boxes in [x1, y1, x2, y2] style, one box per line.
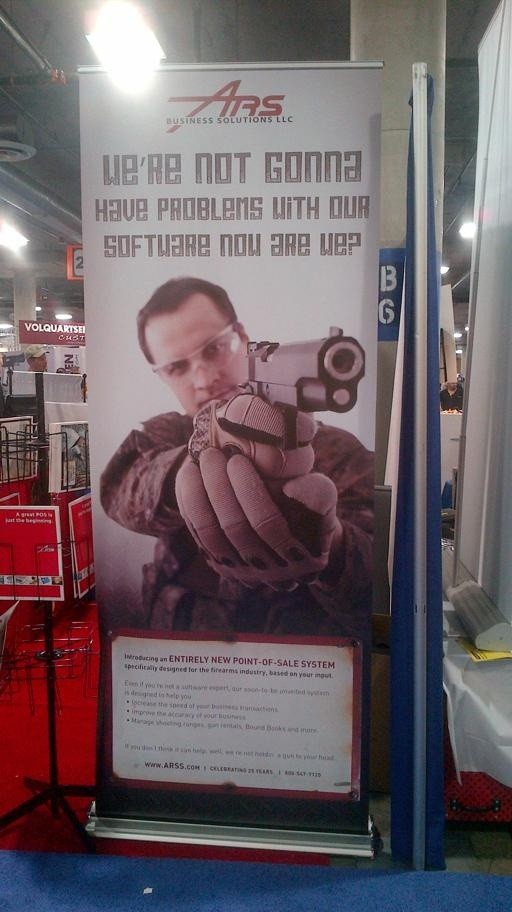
[25, 344, 50, 360]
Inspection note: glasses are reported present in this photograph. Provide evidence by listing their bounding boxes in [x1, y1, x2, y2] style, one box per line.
[150, 325, 241, 383]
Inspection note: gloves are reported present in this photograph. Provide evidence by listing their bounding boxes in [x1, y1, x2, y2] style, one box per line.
[174, 447, 339, 597]
[185, 394, 322, 505]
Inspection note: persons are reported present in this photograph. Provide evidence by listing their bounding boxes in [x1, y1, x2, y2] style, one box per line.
[99, 278, 379, 639]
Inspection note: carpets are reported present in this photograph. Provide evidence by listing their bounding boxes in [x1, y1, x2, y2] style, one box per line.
[2, 830, 511, 911]
[0, 467, 339, 870]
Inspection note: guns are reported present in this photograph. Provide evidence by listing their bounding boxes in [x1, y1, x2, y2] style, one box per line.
[241, 326, 364, 413]
